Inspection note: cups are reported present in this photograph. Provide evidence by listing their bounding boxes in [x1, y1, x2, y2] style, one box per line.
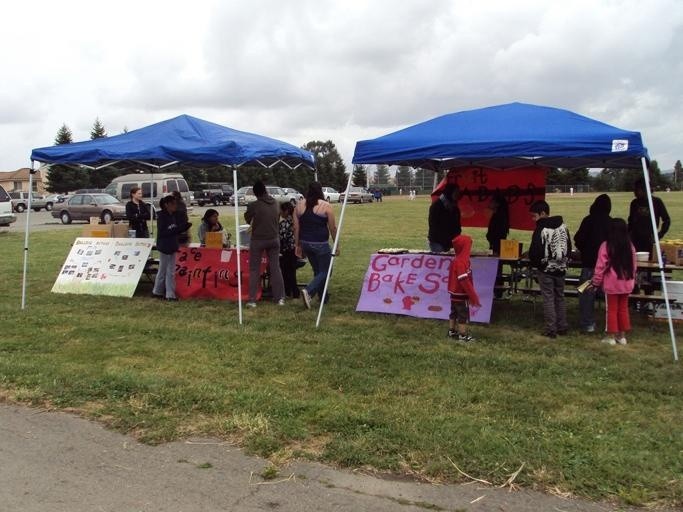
[127, 230, 136, 237]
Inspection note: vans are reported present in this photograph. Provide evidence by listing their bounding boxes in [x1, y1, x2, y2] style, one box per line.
[229, 186, 257, 206]
[104, 172, 193, 220]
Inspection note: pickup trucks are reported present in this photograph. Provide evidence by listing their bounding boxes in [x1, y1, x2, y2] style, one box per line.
[11, 191, 56, 213]
[194, 184, 233, 207]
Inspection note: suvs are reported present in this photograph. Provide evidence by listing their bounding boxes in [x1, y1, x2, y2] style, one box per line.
[0, 183, 16, 227]
[265, 187, 296, 208]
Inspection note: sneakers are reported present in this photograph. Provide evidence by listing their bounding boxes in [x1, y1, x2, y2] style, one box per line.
[276, 298, 285, 305]
[457, 333, 473, 342]
[300, 289, 311, 309]
[602, 336, 615, 347]
[245, 301, 256, 307]
[448, 329, 457, 337]
[615, 338, 627, 344]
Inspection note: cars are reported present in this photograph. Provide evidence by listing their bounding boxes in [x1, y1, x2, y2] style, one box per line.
[46, 195, 63, 203]
[322, 187, 340, 204]
[339, 186, 374, 204]
[51, 193, 126, 224]
[281, 188, 304, 201]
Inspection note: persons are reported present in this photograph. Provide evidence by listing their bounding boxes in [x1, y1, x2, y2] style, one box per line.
[151, 195, 178, 301]
[412, 188, 416, 200]
[408, 188, 413, 200]
[244, 182, 286, 309]
[380, 190, 383, 202]
[400, 187, 402, 195]
[446, 235, 480, 342]
[198, 209, 223, 247]
[292, 181, 339, 310]
[172, 191, 192, 244]
[486, 195, 509, 299]
[428, 183, 462, 253]
[573, 179, 671, 346]
[126, 188, 151, 237]
[375, 190, 381, 202]
[528, 200, 572, 338]
[278, 202, 304, 298]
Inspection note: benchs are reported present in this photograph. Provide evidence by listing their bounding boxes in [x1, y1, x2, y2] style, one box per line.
[494, 283, 512, 303]
[518, 288, 677, 322]
[503, 272, 521, 294]
[533, 274, 671, 286]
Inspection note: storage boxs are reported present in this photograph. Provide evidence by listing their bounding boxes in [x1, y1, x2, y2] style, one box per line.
[499, 239, 523, 259]
[652, 239, 683, 265]
[239, 224, 251, 244]
[113, 221, 131, 238]
[205, 232, 223, 249]
[648, 280, 683, 320]
[81, 223, 114, 238]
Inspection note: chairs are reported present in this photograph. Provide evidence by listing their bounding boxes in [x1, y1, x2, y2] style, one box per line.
[142, 255, 160, 285]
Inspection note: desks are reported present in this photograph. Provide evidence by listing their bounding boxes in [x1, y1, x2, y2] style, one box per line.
[355, 253, 526, 327]
[523, 255, 683, 323]
[174, 243, 270, 303]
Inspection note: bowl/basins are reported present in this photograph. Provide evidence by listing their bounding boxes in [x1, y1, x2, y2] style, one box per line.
[636, 252, 648, 263]
